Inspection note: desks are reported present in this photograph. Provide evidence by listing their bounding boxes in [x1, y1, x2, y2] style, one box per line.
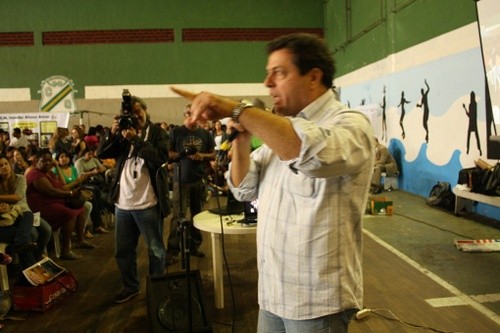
[192, 210, 257, 309]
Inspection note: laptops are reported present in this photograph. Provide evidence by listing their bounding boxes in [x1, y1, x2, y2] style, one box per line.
[208, 190, 245, 215]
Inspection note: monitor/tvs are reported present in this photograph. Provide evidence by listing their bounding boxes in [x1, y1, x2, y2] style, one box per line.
[244, 201, 257, 219]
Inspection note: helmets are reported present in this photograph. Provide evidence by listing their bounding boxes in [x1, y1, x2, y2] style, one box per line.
[239, 97, 264, 111]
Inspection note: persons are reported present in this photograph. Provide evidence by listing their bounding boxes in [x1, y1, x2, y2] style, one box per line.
[168, 31, 377, 333]
[96, 97, 178, 305]
[168, 103, 216, 259]
[0, 114, 265, 286]
[369, 136, 399, 195]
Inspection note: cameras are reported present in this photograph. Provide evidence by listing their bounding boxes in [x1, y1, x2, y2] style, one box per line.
[115, 89, 137, 132]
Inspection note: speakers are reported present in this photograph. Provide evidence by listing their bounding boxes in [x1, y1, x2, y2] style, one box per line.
[146, 269, 214, 333]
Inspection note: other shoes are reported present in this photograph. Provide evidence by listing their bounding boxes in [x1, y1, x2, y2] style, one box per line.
[114, 282, 139, 303]
[188, 249, 205, 256]
[61, 252, 83, 260]
[96, 227, 108, 234]
[84, 231, 95, 238]
[167, 254, 178, 264]
[75, 241, 93, 250]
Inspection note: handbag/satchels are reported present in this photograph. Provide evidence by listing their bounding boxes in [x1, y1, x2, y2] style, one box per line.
[64, 193, 85, 209]
[427, 160, 500, 211]
[87, 167, 104, 184]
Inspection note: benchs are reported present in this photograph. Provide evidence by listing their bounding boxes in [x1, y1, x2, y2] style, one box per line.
[452, 188, 500, 215]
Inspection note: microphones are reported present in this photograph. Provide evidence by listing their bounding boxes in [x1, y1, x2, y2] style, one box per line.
[215, 129, 240, 162]
[160, 147, 198, 168]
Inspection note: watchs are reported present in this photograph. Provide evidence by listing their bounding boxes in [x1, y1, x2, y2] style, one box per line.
[231, 98, 255, 123]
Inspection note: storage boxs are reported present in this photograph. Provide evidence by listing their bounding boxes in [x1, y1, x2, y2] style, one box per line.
[12, 272, 75, 315]
[369, 196, 393, 215]
